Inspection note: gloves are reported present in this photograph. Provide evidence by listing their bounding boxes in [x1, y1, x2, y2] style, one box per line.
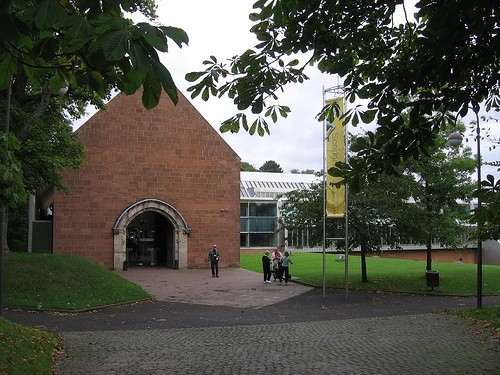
[216, 257, 218, 260]
[208, 261, 211, 264]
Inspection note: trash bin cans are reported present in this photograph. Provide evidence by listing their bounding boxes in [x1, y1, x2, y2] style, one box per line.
[425, 270, 439, 290]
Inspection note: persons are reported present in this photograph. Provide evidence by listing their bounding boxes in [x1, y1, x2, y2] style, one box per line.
[208, 245, 220, 278]
[262, 250, 271, 284]
[271, 248, 293, 285]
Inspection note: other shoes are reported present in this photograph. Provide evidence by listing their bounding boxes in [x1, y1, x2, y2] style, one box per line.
[279, 282, 282, 285]
[210, 276, 215, 278]
[274, 279, 276, 281]
[282, 279, 283, 281]
[216, 276, 220, 278]
[266, 280, 271, 284]
[264, 281, 267, 284]
[284, 282, 288, 285]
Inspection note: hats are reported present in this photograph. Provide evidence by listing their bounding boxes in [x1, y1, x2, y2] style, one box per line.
[265, 250, 269, 253]
[283, 252, 289, 256]
[213, 245, 216, 247]
[273, 247, 279, 251]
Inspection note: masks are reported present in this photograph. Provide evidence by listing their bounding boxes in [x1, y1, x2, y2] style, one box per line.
[213, 248, 216, 250]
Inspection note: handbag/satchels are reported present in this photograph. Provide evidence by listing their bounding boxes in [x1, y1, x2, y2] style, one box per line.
[279, 263, 282, 267]
